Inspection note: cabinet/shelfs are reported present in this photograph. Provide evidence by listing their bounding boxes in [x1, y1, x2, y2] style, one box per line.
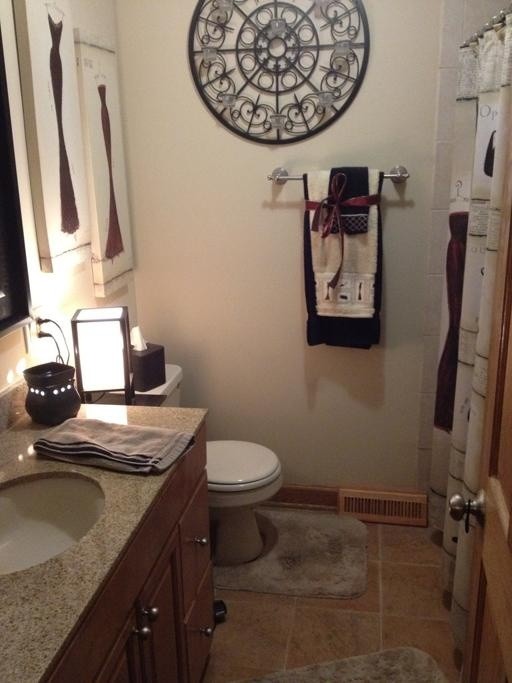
[44, 471, 176, 683]
[177, 421, 218, 683]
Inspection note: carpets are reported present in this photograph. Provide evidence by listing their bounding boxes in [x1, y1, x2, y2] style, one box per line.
[250, 645, 444, 683]
[214, 506, 368, 600]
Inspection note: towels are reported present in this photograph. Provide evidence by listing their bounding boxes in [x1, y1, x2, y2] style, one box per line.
[32, 417, 196, 474]
[303, 165, 386, 350]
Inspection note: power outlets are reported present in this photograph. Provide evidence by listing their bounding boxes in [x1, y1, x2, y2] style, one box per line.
[24, 306, 43, 352]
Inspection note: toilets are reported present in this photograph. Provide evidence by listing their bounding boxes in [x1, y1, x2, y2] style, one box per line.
[91, 362, 283, 566]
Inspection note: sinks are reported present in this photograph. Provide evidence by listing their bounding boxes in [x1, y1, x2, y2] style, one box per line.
[0, 471, 105, 575]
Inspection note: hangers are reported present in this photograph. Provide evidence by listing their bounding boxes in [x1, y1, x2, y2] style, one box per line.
[448, 179, 470, 203]
